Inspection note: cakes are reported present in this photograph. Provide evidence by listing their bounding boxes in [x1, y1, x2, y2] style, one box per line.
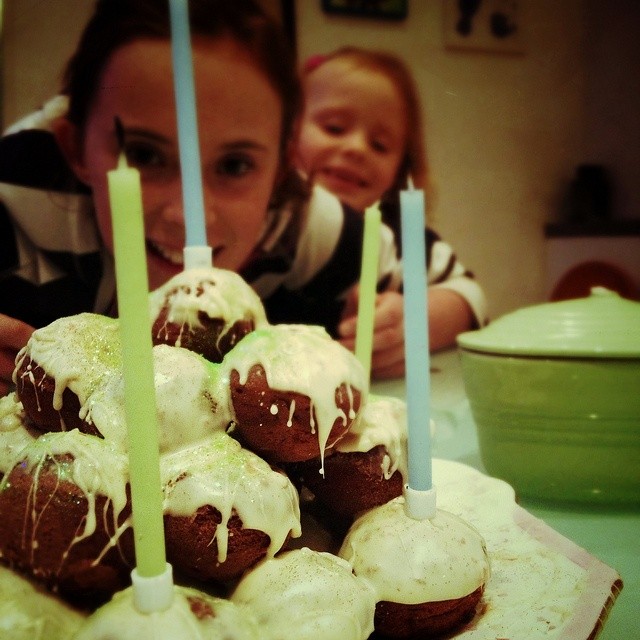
[2, 0, 492, 640]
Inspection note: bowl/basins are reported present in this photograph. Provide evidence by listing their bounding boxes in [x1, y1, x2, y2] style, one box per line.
[454, 287, 640, 508]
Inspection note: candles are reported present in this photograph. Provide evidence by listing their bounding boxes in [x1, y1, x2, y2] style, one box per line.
[164, 3, 216, 271]
[398, 155, 433, 519]
[103, 112, 175, 615]
[351, 200, 383, 412]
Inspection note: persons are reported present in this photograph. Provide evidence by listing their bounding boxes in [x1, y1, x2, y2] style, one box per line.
[290, 44, 488, 381]
[2, 1, 396, 404]
